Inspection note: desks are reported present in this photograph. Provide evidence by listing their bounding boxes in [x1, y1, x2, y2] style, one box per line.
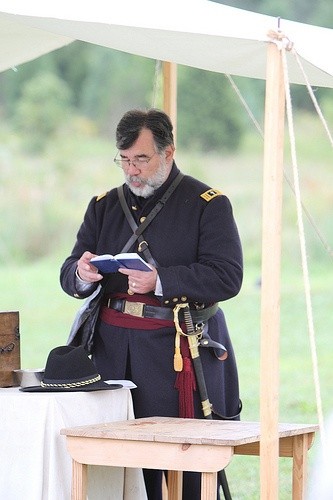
[0, 387, 147, 500]
[60, 417, 319, 500]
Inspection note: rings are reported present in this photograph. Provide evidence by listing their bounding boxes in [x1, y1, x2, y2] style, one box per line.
[132, 282, 136, 288]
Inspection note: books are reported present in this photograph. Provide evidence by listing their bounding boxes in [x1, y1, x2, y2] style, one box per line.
[90, 253, 153, 273]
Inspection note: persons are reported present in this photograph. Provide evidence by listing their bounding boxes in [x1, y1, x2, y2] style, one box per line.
[60, 108, 243, 500]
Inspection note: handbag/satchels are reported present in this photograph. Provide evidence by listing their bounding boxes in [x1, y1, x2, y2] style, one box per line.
[67, 284, 101, 353]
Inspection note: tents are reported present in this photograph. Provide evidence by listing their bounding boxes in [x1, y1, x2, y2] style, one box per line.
[1, 0, 333, 500]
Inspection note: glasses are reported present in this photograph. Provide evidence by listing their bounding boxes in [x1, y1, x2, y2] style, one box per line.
[114, 151, 152, 169]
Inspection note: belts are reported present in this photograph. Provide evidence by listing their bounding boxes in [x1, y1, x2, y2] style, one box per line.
[102, 296, 218, 323]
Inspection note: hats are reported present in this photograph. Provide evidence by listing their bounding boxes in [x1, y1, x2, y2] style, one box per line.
[19, 346, 123, 392]
